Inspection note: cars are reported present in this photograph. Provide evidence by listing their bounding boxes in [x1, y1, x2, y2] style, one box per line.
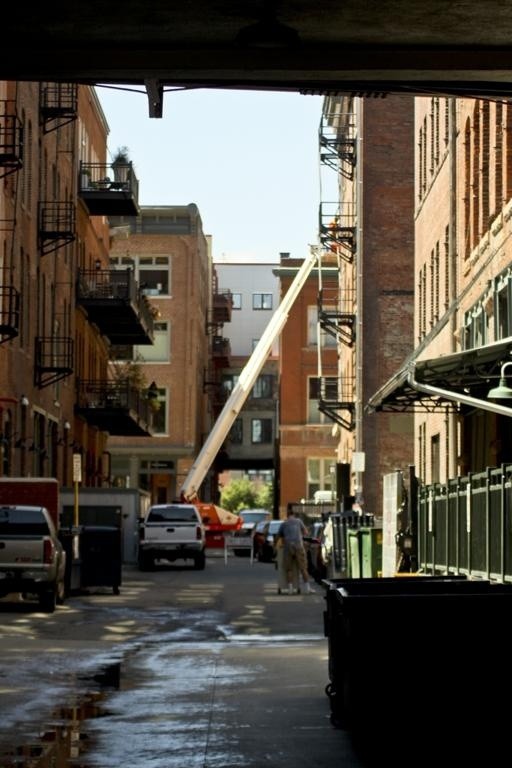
[232, 507, 333, 587]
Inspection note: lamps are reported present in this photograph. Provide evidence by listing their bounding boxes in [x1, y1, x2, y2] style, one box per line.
[486, 361, 511, 399]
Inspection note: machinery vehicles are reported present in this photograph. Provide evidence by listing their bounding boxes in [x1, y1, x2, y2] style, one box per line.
[170, 212, 342, 549]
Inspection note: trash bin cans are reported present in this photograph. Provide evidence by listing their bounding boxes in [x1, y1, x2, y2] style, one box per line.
[321, 575, 512, 768]
[345, 526, 382, 578]
[78, 526, 122, 595]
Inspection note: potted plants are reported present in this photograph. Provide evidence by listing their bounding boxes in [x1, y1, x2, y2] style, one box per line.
[110, 148, 131, 184]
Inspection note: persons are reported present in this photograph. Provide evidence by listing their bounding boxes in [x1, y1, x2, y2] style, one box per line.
[273, 510, 316, 596]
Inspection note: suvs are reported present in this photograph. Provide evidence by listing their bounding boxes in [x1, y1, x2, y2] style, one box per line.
[0, 504, 73, 614]
[135, 502, 207, 570]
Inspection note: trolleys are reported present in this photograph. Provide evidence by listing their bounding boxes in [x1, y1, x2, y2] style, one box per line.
[268, 543, 305, 595]
